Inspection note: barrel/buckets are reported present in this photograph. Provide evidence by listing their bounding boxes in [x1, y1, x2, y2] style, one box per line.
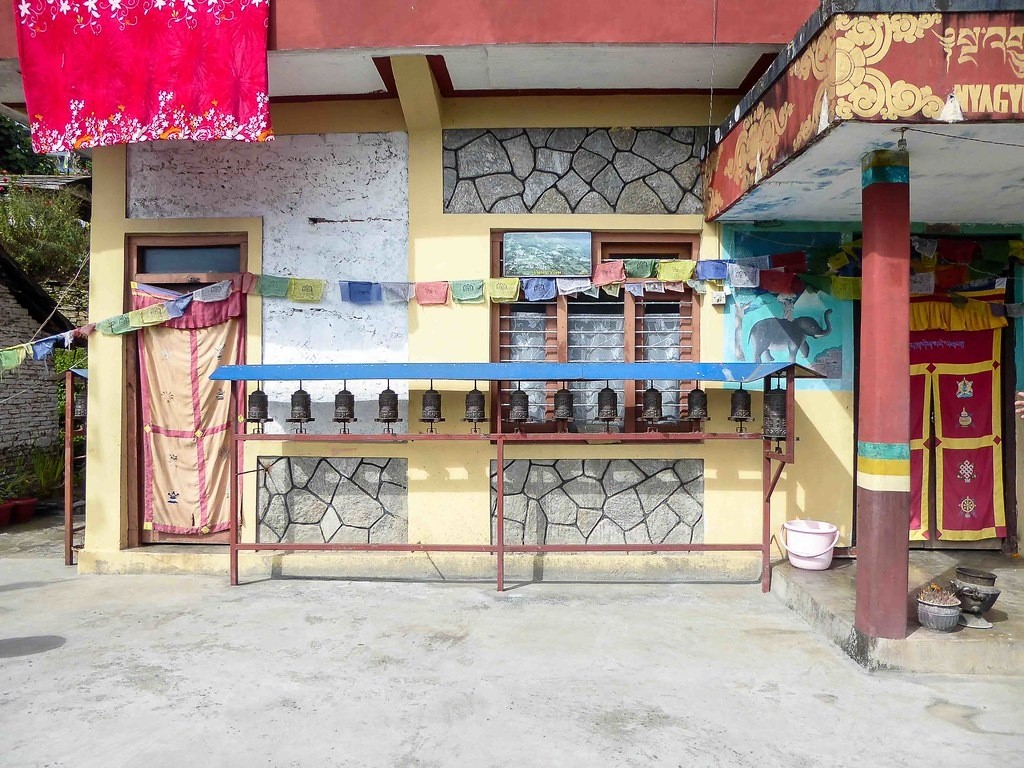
[780, 519, 840, 570]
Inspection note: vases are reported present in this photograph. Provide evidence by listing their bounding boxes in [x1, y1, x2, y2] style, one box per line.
[913, 595, 961, 634]
[948, 567, 1002, 616]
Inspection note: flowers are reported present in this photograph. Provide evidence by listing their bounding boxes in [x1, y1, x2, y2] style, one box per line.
[915, 582, 959, 603]
